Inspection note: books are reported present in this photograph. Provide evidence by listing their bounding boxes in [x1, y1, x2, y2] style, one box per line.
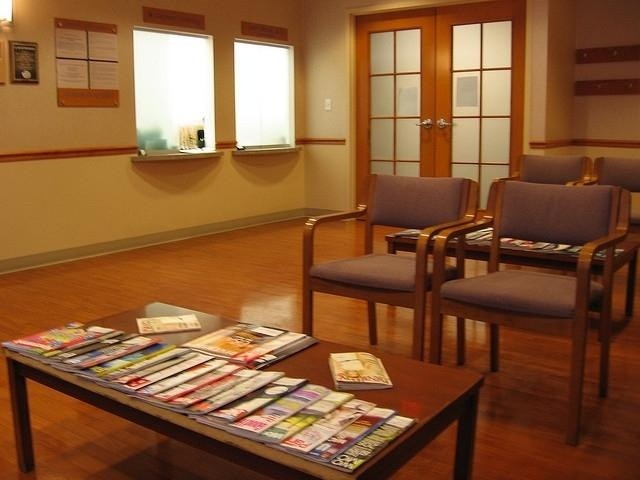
[3, 321, 416, 472]
[137, 315, 201, 334]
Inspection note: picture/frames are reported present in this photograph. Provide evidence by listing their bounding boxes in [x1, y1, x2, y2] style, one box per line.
[8, 39, 40, 83]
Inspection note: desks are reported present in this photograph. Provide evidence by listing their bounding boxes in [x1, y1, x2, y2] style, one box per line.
[0, 296, 484, 480]
[386, 216, 637, 339]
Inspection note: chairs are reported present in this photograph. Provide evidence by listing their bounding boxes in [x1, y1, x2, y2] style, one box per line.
[428, 180, 624, 444]
[303, 172, 480, 359]
[575, 155, 639, 234]
[484, 154, 591, 209]
